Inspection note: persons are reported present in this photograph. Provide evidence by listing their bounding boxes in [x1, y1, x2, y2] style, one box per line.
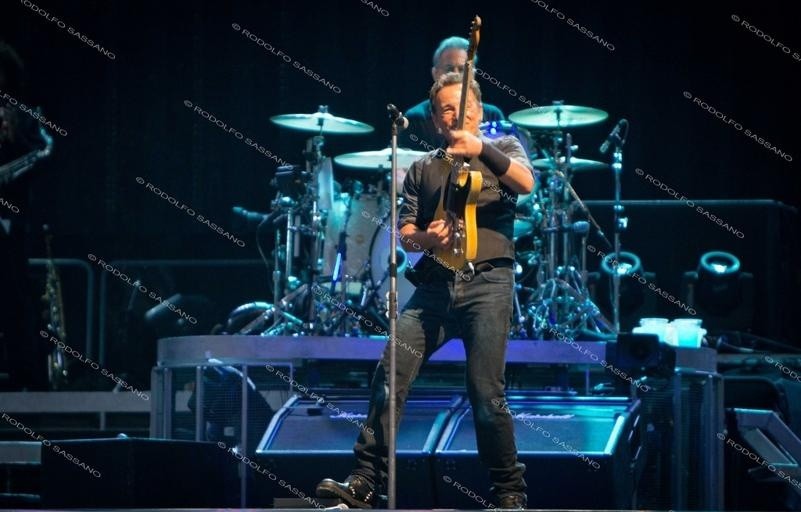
[395, 35, 506, 153]
[314, 73, 539, 508]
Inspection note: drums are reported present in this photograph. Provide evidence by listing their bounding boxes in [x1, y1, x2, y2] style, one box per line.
[368, 203, 428, 331]
[318, 194, 392, 295]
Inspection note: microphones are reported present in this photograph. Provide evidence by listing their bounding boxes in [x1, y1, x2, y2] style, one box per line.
[601, 124, 622, 154]
[387, 247, 406, 269]
[387, 103, 408, 128]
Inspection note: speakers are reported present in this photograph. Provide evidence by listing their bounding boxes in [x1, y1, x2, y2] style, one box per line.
[437, 399, 646, 512]
[255, 393, 463, 507]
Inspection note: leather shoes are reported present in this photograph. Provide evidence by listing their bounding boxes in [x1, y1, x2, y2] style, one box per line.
[316, 473, 387, 509]
[497, 493, 526, 509]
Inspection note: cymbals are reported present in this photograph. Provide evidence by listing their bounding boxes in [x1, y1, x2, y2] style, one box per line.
[335, 147, 428, 170]
[532, 156, 607, 171]
[509, 103, 607, 128]
[271, 113, 373, 135]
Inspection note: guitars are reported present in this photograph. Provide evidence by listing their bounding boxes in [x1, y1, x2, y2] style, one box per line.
[423, 16, 484, 274]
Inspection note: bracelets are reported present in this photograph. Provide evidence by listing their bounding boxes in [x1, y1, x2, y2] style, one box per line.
[480, 138, 511, 177]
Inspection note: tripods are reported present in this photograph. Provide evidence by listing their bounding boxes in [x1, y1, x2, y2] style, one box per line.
[236, 127, 389, 332]
[512, 130, 611, 341]
